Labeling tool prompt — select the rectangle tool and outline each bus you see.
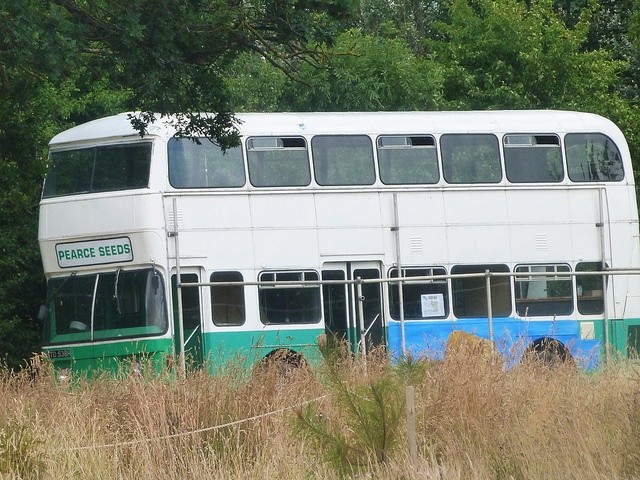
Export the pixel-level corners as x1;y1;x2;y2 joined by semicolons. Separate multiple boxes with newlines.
37;109;640;399
